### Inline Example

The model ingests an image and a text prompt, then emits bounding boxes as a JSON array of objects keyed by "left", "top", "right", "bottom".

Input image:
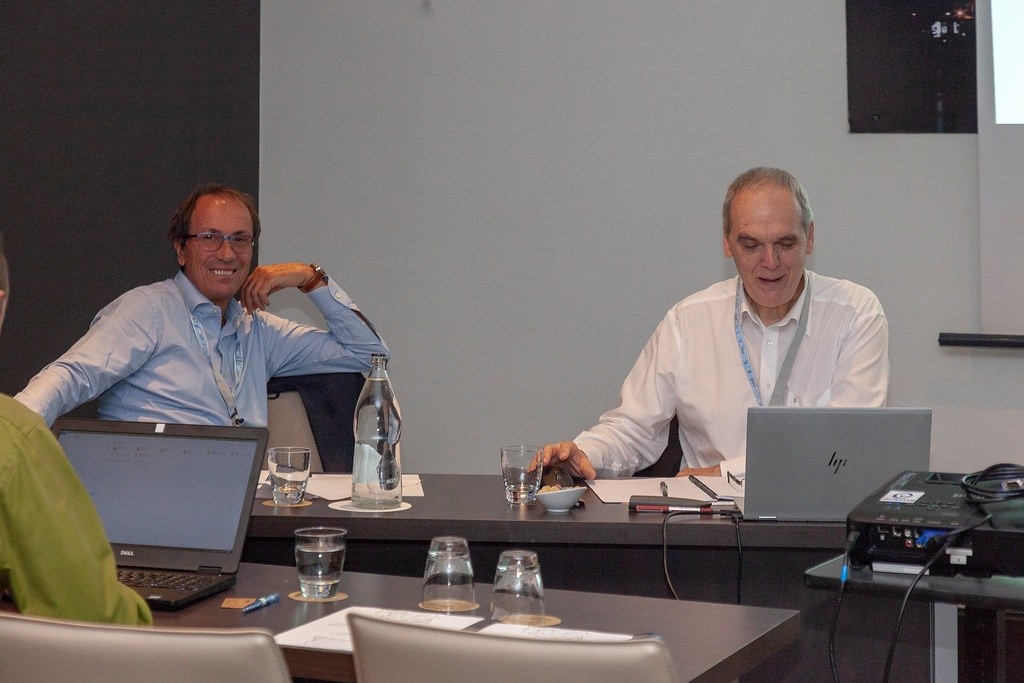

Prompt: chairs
[
  {"left": 0, "top": 610, "right": 294, "bottom": 683},
  {"left": 347, "top": 613, "right": 677, "bottom": 683}
]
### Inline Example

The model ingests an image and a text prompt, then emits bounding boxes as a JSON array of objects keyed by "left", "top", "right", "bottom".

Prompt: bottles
[{"left": 351, "top": 353, "right": 402, "bottom": 509}]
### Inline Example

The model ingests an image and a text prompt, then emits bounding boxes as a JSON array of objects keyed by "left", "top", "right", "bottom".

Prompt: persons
[
  {"left": 527, "top": 166, "right": 890, "bottom": 480},
  {"left": 14, "top": 183, "right": 391, "bottom": 426},
  {"left": 0, "top": 251, "right": 152, "bottom": 626}
]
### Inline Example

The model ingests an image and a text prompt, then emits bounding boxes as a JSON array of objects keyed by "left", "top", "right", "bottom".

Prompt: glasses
[{"left": 180, "top": 232, "right": 256, "bottom": 253}]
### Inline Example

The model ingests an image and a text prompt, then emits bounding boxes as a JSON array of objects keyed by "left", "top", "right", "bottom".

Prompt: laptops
[
  {"left": 734, "top": 407, "right": 933, "bottom": 521},
  {"left": 0, "top": 417, "right": 270, "bottom": 613}
]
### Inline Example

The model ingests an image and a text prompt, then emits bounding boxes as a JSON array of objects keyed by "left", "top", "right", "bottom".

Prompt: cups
[
  {"left": 500, "top": 445, "right": 544, "bottom": 504},
  {"left": 423, "top": 537, "right": 474, "bottom": 611},
  {"left": 266, "top": 447, "right": 311, "bottom": 506},
  {"left": 294, "top": 526, "right": 347, "bottom": 599},
  {"left": 490, "top": 549, "right": 544, "bottom": 626}
]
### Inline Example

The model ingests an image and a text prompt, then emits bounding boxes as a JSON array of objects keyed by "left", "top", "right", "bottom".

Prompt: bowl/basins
[{"left": 533, "top": 487, "right": 588, "bottom": 512}]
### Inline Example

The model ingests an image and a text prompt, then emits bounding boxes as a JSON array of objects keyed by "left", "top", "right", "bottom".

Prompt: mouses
[{"left": 532, "top": 465, "right": 574, "bottom": 489}]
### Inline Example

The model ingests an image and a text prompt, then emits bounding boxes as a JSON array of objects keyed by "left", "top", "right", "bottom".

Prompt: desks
[{"left": 149, "top": 472, "right": 1024, "bottom": 683}]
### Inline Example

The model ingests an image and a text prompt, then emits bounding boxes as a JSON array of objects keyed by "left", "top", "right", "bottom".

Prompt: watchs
[{"left": 302, "top": 263, "right": 326, "bottom": 293}]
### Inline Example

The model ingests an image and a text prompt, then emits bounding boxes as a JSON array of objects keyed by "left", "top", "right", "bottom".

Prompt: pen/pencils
[
  {"left": 660, "top": 481, "right": 668, "bottom": 497},
  {"left": 242, "top": 592, "right": 280, "bottom": 614},
  {"left": 689, "top": 475, "right": 720, "bottom": 501},
  {"left": 636, "top": 505, "right": 712, "bottom": 513}
]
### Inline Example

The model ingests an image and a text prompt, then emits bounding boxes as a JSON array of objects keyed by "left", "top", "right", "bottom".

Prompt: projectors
[{"left": 847, "top": 471, "right": 1024, "bottom": 580}]
